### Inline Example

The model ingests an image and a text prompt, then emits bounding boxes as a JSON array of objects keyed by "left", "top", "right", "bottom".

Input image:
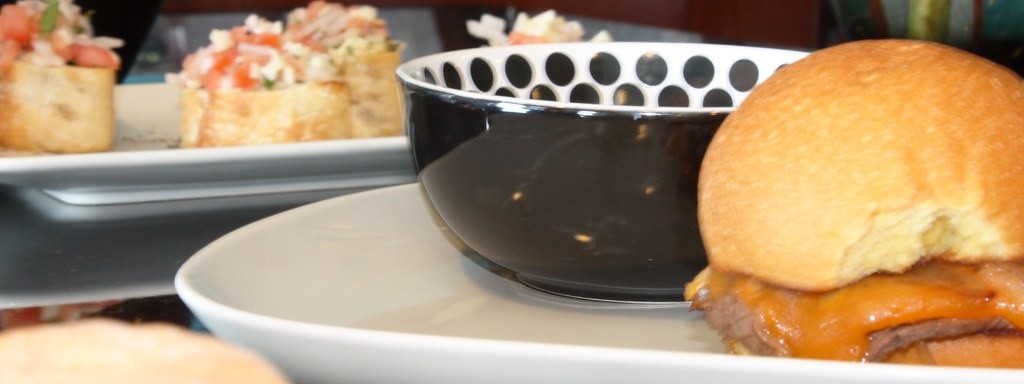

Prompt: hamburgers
[{"left": 682, "top": 38, "right": 1024, "bottom": 374}]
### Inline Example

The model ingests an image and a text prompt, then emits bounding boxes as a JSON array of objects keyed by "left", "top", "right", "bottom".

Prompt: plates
[
  {"left": 122, "top": 9, "right": 444, "bottom": 91},
  {"left": 173, "top": 183, "right": 1024, "bottom": 384},
  {"left": 0, "top": 16, "right": 708, "bottom": 206}
]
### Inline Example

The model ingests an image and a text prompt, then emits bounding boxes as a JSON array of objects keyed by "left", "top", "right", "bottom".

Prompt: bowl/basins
[{"left": 394, "top": 41, "right": 812, "bottom": 305}]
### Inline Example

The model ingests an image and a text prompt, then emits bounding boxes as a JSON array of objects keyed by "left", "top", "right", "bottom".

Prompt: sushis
[{"left": 0, "top": 0, "right": 617, "bottom": 151}]
